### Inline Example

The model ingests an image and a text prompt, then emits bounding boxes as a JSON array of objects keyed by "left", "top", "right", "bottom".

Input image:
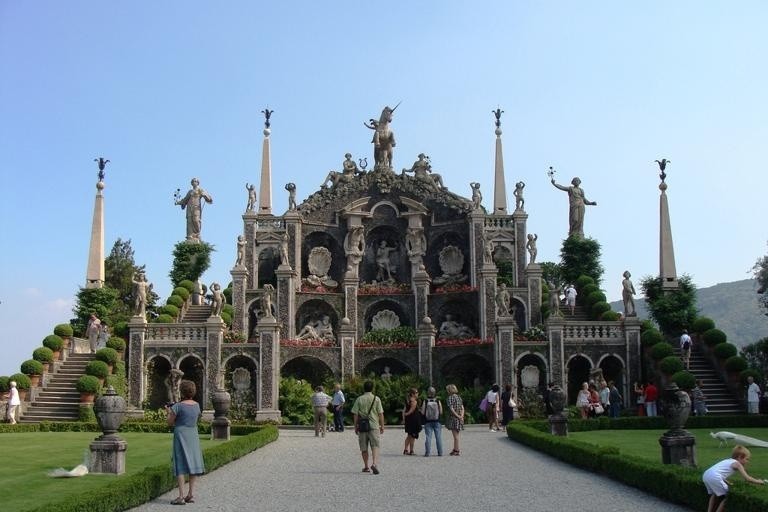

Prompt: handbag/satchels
[
  {"left": 593, "top": 404, "right": 605, "bottom": 414},
  {"left": 357, "top": 417, "right": 370, "bottom": 433},
  {"left": 508, "top": 392, "right": 517, "bottom": 407},
  {"left": 479, "top": 398, "right": 489, "bottom": 412}
]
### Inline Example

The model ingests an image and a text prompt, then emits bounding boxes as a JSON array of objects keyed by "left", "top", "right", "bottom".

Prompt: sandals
[
  {"left": 170, "top": 495, "right": 195, "bottom": 505},
  {"left": 362, "top": 464, "right": 380, "bottom": 474},
  {"left": 449, "top": 450, "right": 461, "bottom": 456},
  {"left": 403, "top": 450, "right": 415, "bottom": 455}
]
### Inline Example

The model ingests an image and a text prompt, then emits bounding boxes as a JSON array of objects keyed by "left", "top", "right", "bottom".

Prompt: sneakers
[{"left": 489, "top": 427, "right": 507, "bottom": 433}]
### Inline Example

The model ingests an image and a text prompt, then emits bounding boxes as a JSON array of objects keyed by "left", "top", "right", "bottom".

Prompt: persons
[
  {"left": 376, "top": 241, "right": 397, "bottom": 280},
  {"left": 440, "top": 315, "right": 474, "bottom": 337},
  {"left": 403, "top": 154, "right": 448, "bottom": 195},
  {"left": 513, "top": 181, "right": 525, "bottom": 210},
  {"left": 495, "top": 284, "right": 511, "bottom": 317},
  {"left": 86, "top": 314, "right": 101, "bottom": 354},
  {"left": 747, "top": 376, "right": 761, "bottom": 415},
  {"left": 176, "top": 178, "right": 212, "bottom": 239},
  {"left": 482, "top": 230, "right": 494, "bottom": 263},
  {"left": 622, "top": 271, "right": 636, "bottom": 316},
  {"left": 131, "top": 272, "right": 149, "bottom": 317},
  {"left": 285, "top": 183, "right": 297, "bottom": 212},
  {"left": 3, "top": 382, "right": 20, "bottom": 425},
  {"left": 363, "top": 120, "right": 379, "bottom": 143},
  {"left": 99, "top": 321, "right": 111, "bottom": 349},
  {"left": 702, "top": 445, "right": 765, "bottom": 512},
  {"left": 164, "top": 369, "right": 185, "bottom": 404},
  {"left": 278, "top": 234, "right": 290, "bottom": 265},
  {"left": 499, "top": 383, "right": 517, "bottom": 431},
  {"left": 544, "top": 382, "right": 555, "bottom": 419},
  {"left": 296, "top": 316, "right": 332, "bottom": 340},
  {"left": 526, "top": 234, "right": 537, "bottom": 263},
  {"left": 164, "top": 380, "right": 205, "bottom": 505},
  {"left": 262, "top": 284, "right": 274, "bottom": 317},
  {"left": 484, "top": 383, "right": 500, "bottom": 432},
  {"left": 576, "top": 379, "right": 658, "bottom": 420},
  {"left": 551, "top": 177, "right": 597, "bottom": 237},
  {"left": 679, "top": 329, "right": 693, "bottom": 370},
  {"left": 312, "top": 380, "right": 464, "bottom": 474},
  {"left": 209, "top": 282, "right": 226, "bottom": 316},
  {"left": 235, "top": 235, "right": 247, "bottom": 267},
  {"left": 693, "top": 379, "right": 707, "bottom": 417},
  {"left": 246, "top": 183, "right": 257, "bottom": 212},
  {"left": 562, "top": 281, "right": 577, "bottom": 317},
  {"left": 320, "top": 153, "right": 365, "bottom": 190},
  {"left": 470, "top": 183, "right": 482, "bottom": 209},
  {"left": 381, "top": 366, "right": 392, "bottom": 386},
  {"left": 548, "top": 284, "right": 562, "bottom": 316}
]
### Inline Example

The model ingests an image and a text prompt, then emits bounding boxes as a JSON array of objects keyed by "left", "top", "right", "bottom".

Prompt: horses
[{"left": 373, "top": 106, "right": 396, "bottom": 169}]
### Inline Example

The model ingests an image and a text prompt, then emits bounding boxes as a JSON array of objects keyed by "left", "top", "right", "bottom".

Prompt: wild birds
[{"left": 709, "top": 431, "right": 768, "bottom": 448}]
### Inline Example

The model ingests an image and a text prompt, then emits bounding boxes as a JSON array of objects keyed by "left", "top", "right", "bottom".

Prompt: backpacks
[
  {"left": 425, "top": 398, "right": 440, "bottom": 422},
  {"left": 683, "top": 341, "right": 690, "bottom": 350}
]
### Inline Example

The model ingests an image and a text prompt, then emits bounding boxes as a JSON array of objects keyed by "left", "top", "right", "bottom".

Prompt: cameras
[{"left": 164, "top": 402, "right": 175, "bottom": 409}]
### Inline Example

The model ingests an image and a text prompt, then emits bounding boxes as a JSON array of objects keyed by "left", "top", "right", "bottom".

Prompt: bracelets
[{"left": 167, "top": 413, "right": 170, "bottom": 414}]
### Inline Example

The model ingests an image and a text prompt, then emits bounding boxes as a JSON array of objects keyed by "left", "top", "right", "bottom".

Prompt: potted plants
[
  {"left": 592, "top": 301, "right": 745, "bottom": 399},
  {"left": 0, "top": 314, "right": 173, "bottom": 403}
]
